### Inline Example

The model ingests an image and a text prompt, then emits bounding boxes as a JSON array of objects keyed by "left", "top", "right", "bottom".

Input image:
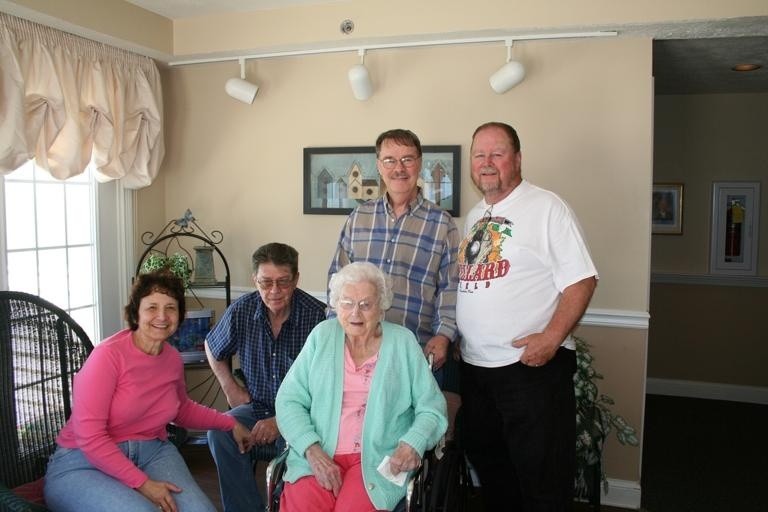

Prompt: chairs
[
  {"left": 0, "top": 291, "right": 187, "bottom": 512},
  {"left": 229, "top": 369, "right": 280, "bottom": 475}
]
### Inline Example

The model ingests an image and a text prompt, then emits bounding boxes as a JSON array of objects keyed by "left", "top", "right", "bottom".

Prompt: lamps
[
  {"left": 349, "top": 49, "right": 373, "bottom": 100},
  {"left": 489, "top": 40, "right": 526, "bottom": 94},
  {"left": 224, "top": 59, "right": 259, "bottom": 105}
]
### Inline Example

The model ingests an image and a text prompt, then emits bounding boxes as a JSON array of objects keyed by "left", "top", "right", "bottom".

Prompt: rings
[{"left": 262, "top": 436, "right": 270, "bottom": 442}]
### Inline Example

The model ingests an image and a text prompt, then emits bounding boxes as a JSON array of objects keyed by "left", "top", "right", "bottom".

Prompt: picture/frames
[
  {"left": 303, "top": 145, "right": 461, "bottom": 217},
  {"left": 708, "top": 181, "right": 761, "bottom": 276},
  {"left": 652, "top": 182, "right": 684, "bottom": 236}
]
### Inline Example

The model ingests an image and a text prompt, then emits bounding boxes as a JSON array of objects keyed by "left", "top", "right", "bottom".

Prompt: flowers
[{"left": 139, "top": 252, "right": 206, "bottom": 308}]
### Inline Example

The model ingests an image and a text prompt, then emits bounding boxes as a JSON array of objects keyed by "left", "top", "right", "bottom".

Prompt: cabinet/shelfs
[{"left": 131, "top": 208, "right": 231, "bottom": 452}]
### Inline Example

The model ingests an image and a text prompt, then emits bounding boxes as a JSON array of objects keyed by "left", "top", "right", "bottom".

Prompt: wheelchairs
[{"left": 266, "top": 353, "right": 436, "bottom": 512}]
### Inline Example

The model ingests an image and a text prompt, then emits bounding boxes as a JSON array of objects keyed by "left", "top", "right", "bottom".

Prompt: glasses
[
  {"left": 377, "top": 156, "right": 422, "bottom": 169},
  {"left": 256, "top": 279, "right": 296, "bottom": 289},
  {"left": 337, "top": 296, "right": 376, "bottom": 311}
]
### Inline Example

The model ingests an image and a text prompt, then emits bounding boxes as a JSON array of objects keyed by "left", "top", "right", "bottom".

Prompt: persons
[
  {"left": 46, "top": 273, "right": 255, "bottom": 511},
  {"left": 458, "top": 122, "right": 598, "bottom": 512},
  {"left": 205, "top": 243, "right": 326, "bottom": 511},
  {"left": 275, "top": 261, "right": 447, "bottom": 512},
  {"left": 323, "top": 129, "right": 456, "bottom": 373}
]
